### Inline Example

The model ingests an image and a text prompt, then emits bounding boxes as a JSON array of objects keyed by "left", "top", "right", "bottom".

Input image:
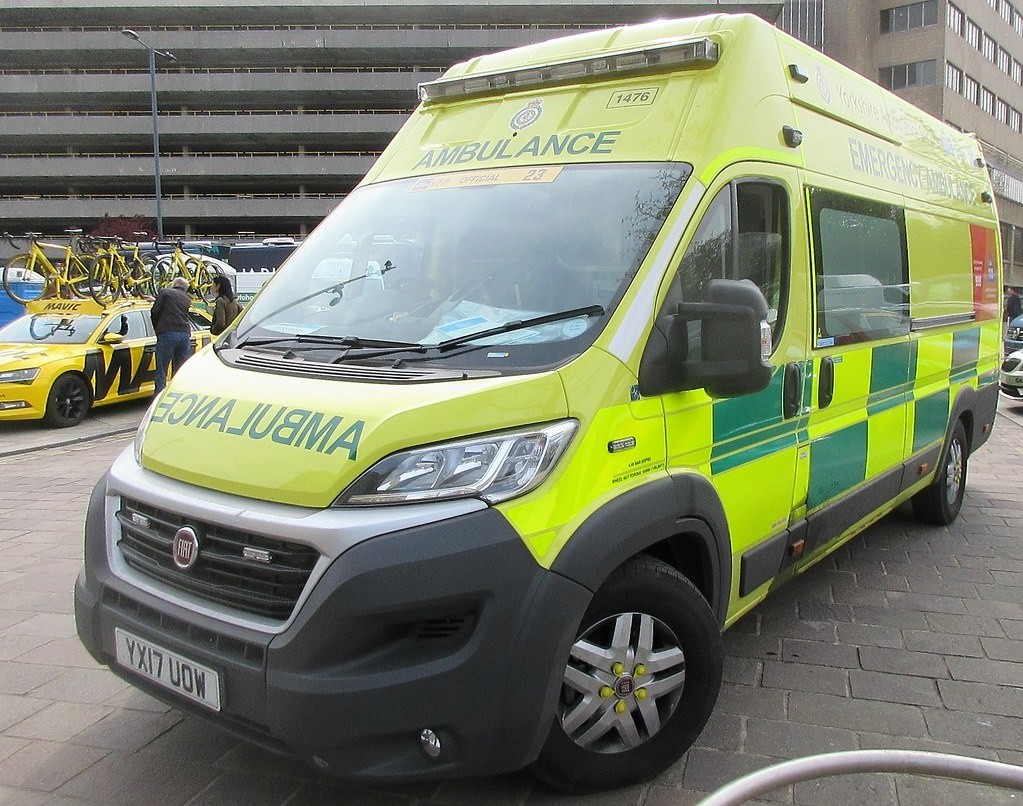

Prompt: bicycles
[{"left": 0, "top": 229, "right": 224, "bottom": 306}]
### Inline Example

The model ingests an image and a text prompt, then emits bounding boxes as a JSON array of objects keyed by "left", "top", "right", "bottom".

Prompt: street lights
[{"left": 123, "top": 30, "right": 177, "bottom": 243}]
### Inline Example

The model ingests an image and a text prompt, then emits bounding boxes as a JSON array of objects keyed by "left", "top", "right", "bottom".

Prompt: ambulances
[{"left": 74, "top": 12, "right": 1004, "bottom": 791}]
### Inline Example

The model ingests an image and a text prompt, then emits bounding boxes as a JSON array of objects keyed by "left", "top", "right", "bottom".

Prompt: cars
[{"left": 0, "top": 304, "right": 222, "bottom": 428}]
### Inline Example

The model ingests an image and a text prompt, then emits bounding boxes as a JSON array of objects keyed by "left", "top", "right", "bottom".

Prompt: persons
[
  {"left": 143, "top": 277, "right": 192, "bottom": 409},
  {"left": 210, "top": 276, "right": 244, "bottom": 341},
  {"left": 1006, "top": 287, "right": 1022, "bottom": 326}
]
